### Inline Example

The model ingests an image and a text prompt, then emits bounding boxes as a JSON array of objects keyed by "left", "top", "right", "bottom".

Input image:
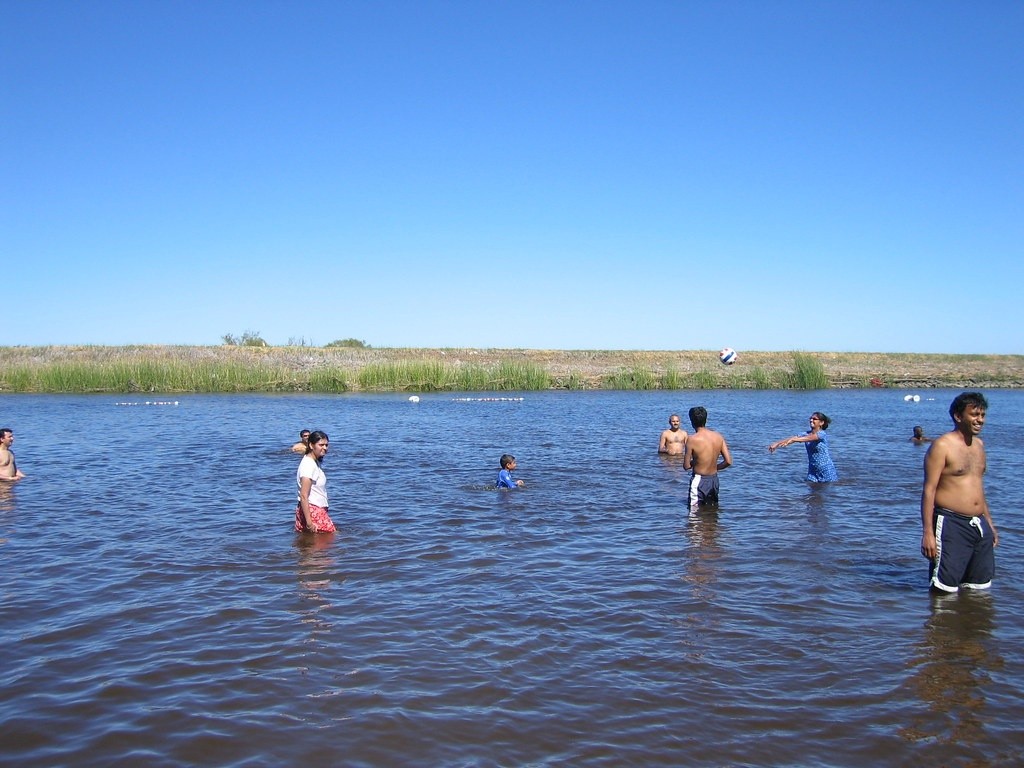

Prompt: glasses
[{"left": 810, "top": 417, "right": 821, "bottom": 420}]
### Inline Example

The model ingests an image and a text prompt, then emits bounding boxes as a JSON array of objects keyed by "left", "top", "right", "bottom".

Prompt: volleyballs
[{"left": 719, "top": 348, "right": 737, "bottom": 365}]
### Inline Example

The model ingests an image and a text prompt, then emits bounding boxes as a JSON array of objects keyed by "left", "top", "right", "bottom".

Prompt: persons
[
  {"left": 0, "top": 428, "right": 25, "bottom": 481},
  {"left": 681, "top": 406, "right": 732, "bottom": 512},
  {"left": 921, "top": 391, "right": 999, "bottom": 592},
  {"left": 294, "top": 429, "right": 335, "bottom": 534},
  {"left": 497, "top": 454, "right": 524, "bottom": 489},
  {"left": 658, "top": 414, "right": 688, "bottom": 454},
  {"left": 769, "top": 411, "right": 839, "bottom": 483},
  {"left": 909, "top": 425, "right": 935, "bottom": 444},
  {"left": 292, "top": 429, "right": 310, "bottom": 454}
]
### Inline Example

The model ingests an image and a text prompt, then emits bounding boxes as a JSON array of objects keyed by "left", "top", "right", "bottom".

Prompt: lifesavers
[{"left": 871, "top": 378, "right": 881, "bottom": 387}]
